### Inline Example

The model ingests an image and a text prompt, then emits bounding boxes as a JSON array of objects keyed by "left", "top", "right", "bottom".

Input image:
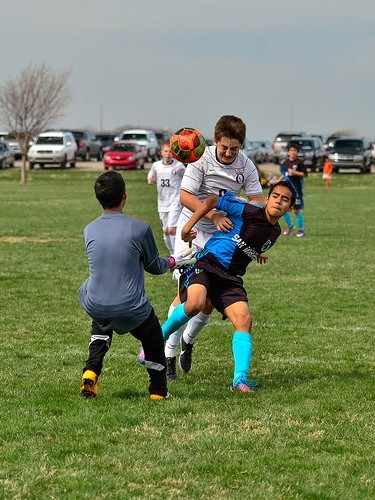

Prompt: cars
[
  {"left": 0, "top": 131, "right": 22, "bottom": 161},
  {"left": 103, "top": 141, "right": 145, "bottom": 171},
  {"left": 91, "top": 133, "right": 116, "bottom": 149},
  {"left": 0, "top": 140, "right": 15, "bottom": 169}
]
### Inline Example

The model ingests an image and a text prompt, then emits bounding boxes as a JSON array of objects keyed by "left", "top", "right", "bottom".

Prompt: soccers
[{"left": 170, "top": 127, "right": 206, "bottom": 163}]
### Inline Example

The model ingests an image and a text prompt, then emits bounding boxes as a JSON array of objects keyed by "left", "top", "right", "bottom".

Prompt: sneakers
[
  {"left": 296, "top": 231, "right": 305, "bottom": 237},
  {"left": 179, "top": 335, "right": 194, "bottom": 372},
  {"left": 283, "top": 226, "right": 296, "bottom": 236},
  {"left": 165, "top": 356, "right": 178, "bottom": 380},
  {"left": 150, "top": 391, "right": 172, "bottom": 400},
  {"left": 139, "top": 348, "right": 145, "bottom": 364},
  {"left": 229, "top": 382, "right": 254, "bottom": 393}
]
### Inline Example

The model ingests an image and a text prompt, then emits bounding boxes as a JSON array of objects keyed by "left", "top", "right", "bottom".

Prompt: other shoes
[{"left": 81, "top": 370, "right": 97, "bottom": 398}]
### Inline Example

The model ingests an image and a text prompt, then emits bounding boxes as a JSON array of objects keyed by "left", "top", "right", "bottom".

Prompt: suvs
[
  {"left": 271, "top": 132, "right": 308, "bottom": 165},
  {"left": 114, "top": 129, "right": 158, "bottom": 163},
  {"left": 70, "top": 130, "right": 104, "bottom": 163},
  {"left": 26, "top": 131, "right": 79, "bottom": 170},
  {"left": 326, "top": 137, "right": 372, "bottom": 174},
  {"left": 279, "top": 137, "right": 325, "bottom": 174},
  {"left": 153, "top": 132, "right": 166, "bottom": 150}
]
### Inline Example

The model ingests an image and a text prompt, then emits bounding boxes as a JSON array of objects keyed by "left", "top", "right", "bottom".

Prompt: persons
[
  {"left": 322, "top": 156, "right": 333, "bottom": 188},
  {"left": 275, "top": 146, "right": 307, "bottom": 238},
  {"left": 148, "top": 140, "right": 186, "bottom": 278},
  {"left": 76, "top": 171, "right": 196, "bottom": 401},
  {"left": 164, "top": 116, "right": 264, "bottom": 378},
  {"left": 138, "top": 180, "right": 297, "bottom": 393}
]
